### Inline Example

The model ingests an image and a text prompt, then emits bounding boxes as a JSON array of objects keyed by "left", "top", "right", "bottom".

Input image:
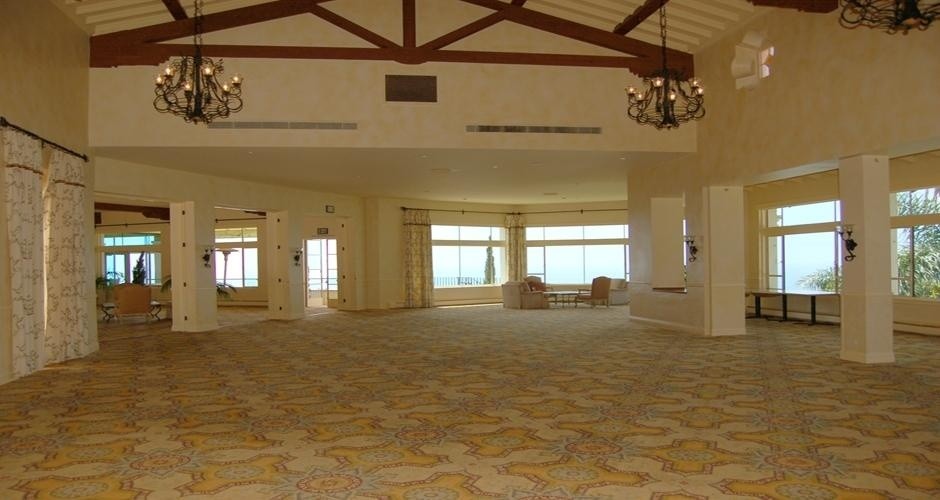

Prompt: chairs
[
  {"left": 112, "top": 285, "right": 151, "bottom": 326},
  {"left": 576, "top": 275, "right": 611, "bottom": 310},
  {"left": 501, "top": 279, "right": 547, "bottom": 309},
  {"left": 608, "top": 279, "right": 629, "bottom": 305},
  {"left": 524, "top": 277, "right": 558, "bottom": 303}
]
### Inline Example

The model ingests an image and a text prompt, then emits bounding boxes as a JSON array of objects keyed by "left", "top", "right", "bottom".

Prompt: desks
[
  {"left": 544, "top": 291, "right": 588, "bottom": 308},
  {"left": 101, "top": 300, "right": 162, "bottom": 325},
  {"left": 749, "top": 290, "right": 838, "bottom": 327}
]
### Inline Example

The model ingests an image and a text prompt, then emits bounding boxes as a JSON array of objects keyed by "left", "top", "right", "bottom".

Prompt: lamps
[
  {"left": 626, "top": 0, "right": 707, "bottom": 130},
  {"left": 682, "top": 235, "right": 698, "bottom": 262},
  {"left": 835, "top": 222, "right": 860, "bottom": 262},
  {"left": 149, "top": 0, "right": 244, "bottom": 125},
  {"left": 832, "top": 1, "right": 940, "bottom": 38}
]
